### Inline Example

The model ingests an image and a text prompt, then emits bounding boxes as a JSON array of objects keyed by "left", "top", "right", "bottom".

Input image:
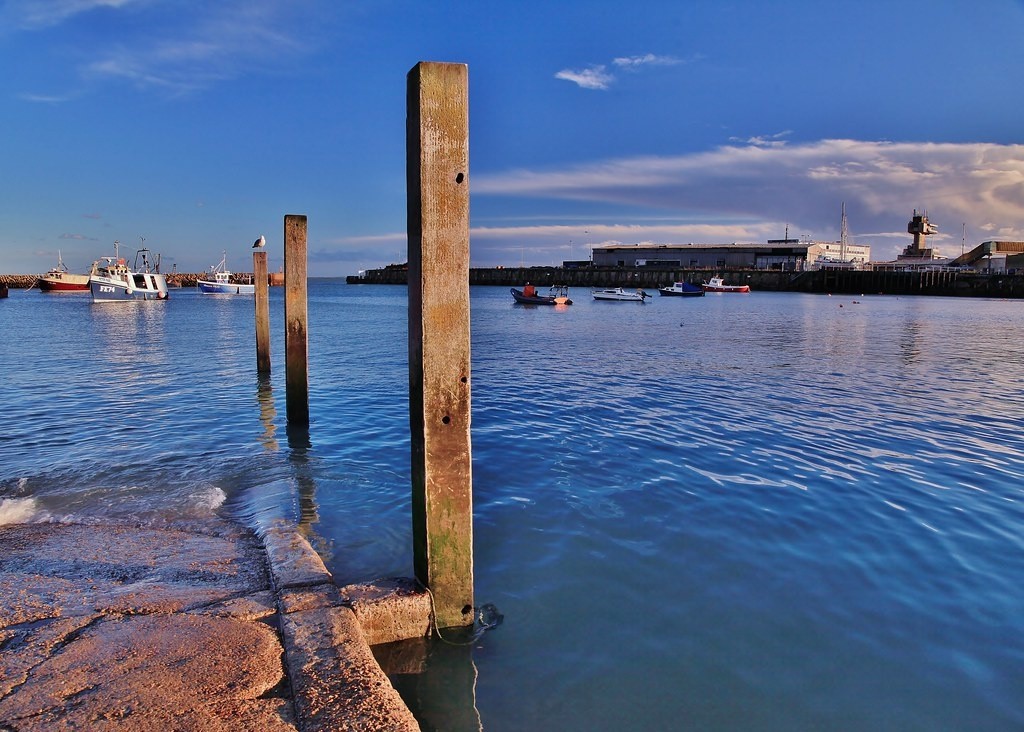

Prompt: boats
[
  {"left": 89, "top": 236, "right": 171, "bottom": 302},
  {"left": 510, "top": 285, "right": 573, "bottom": 306},
  {"left": 658, "top": 281, "right": 705, "bottom": 296},
  {"left": 0, "top": 280, "right": 8, "bottom": 298},
  {"left": 196, "top": 250, "right": 269, "bottom": 294},
  {"left": 590, "top": 287, "right": 652, "bottom": 301},
  {"left": 36, "top": 250, "right": 91, "bottom": 293},
  {"left": 702, "top": 276, "right": 750, "bottom": 292}
]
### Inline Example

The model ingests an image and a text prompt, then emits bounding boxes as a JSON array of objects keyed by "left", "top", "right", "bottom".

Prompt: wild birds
[{"left": 252, "top": 234, "right": 265, "bottom": 251}]
